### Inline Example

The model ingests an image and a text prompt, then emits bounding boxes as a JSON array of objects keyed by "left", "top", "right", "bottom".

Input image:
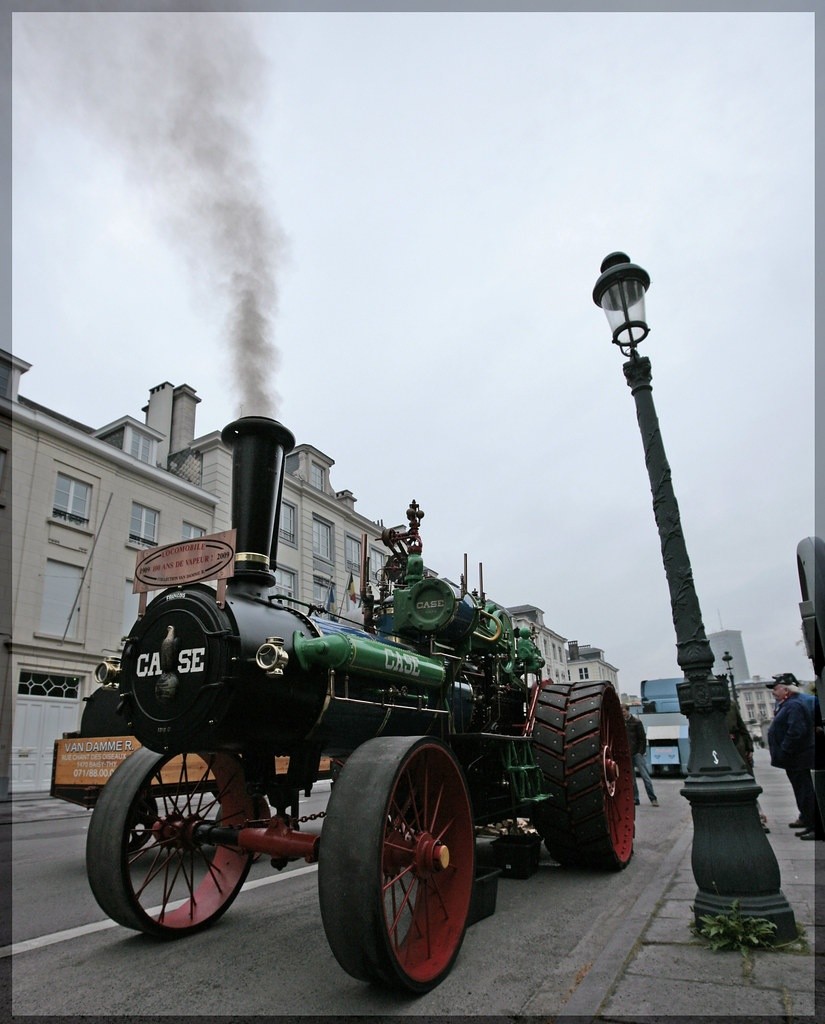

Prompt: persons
[
  {"left": 725, "top": 698, "right": 771, "bottom": 834},
  {"left": 621, "top": 703, "right": 659, "bottom": 807},
  {"left": 765, "top": 672, "right": 823, "bottom": 840}
]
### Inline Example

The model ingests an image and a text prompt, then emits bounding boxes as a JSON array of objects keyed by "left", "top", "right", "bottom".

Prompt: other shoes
[
  {"left": 761, "top": 823, "right": 769, "bottom": 833},
  {"left": 634, "top": 799, "right": 640, "bottom": 804},
  {"left": 789, "top": 819, "right": 806, "bottom": 828},
  {"left": 795, "top": 829, "right": 812, "bottom": 836},
  {"left": 801, "top": 831, "right": 819, "bottom": 840},
  {"left": 651, "top": 799, "right": 659, "bottom": 807}
]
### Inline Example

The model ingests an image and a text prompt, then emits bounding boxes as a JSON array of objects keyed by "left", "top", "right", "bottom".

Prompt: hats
[
  {"left": 766, "top": 677, "right": 791, "bottom": 689},
  {"left": 772, "top": 672, "right": 800, "bottom": 686}
]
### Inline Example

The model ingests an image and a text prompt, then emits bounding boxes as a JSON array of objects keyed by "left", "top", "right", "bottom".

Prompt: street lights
[
  {"left": 598, "top": 252, "right": 805, "bottom": 949},
  {"left": 720, "top": 650, "right": 742, "bottom": 714}
]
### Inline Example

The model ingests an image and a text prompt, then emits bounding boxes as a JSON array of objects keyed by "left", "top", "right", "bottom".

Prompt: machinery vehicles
[
  {"left": 627, "top": 678, "right": 689, "bottom": 778},
  {"left": 49, "top": 411, "right": 639, "bottom": 998}
]
